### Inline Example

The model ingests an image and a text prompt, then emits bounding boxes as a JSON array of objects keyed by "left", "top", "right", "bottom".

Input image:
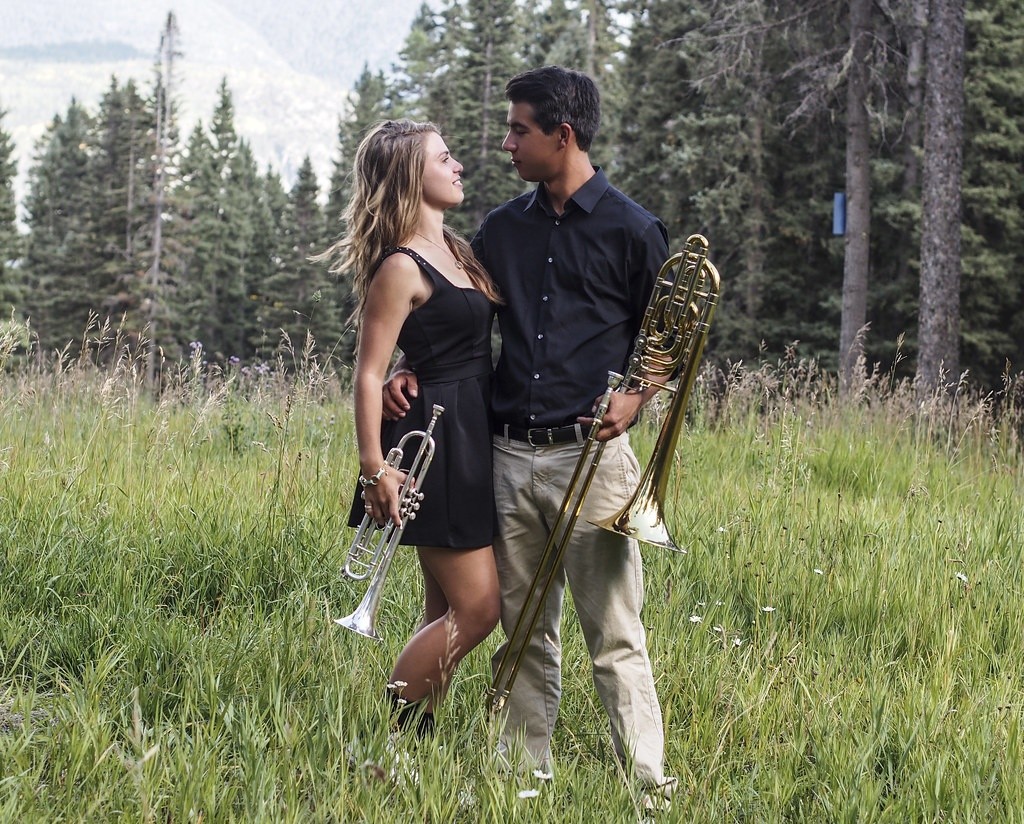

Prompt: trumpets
[{"left": 332, "top": 402, "right": 446, "bottom": 642}]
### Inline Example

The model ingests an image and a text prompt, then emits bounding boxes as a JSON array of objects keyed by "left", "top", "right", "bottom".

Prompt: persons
[
  {"left": 381, "top": 62, "right": 678, "bottom": 824},
  {"left": 302, "top": 117, "right": 504, "bottom": 746}
]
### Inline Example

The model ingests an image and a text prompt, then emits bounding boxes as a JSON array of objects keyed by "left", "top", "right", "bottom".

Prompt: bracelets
[{"left": 359, "top": 460, "right": 389, "bottom": 488}]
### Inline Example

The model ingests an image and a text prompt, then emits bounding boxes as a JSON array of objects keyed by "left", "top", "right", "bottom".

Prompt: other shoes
[{"left": 386, "top": 681, "right": 438, "bottom": 757}]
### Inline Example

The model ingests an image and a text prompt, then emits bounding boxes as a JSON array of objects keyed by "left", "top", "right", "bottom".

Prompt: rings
[{"left": 365, "top": 505, "right": 372, "bottom": 509}]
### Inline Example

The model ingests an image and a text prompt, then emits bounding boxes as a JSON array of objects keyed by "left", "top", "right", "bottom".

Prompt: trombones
[{"left": 486, "top": 233, "right": 722, "bottom": 734}]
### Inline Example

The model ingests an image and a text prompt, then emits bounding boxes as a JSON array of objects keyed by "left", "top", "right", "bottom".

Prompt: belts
[{"left": 493, "top": 421, "right": 591, "bottom": 446}]
